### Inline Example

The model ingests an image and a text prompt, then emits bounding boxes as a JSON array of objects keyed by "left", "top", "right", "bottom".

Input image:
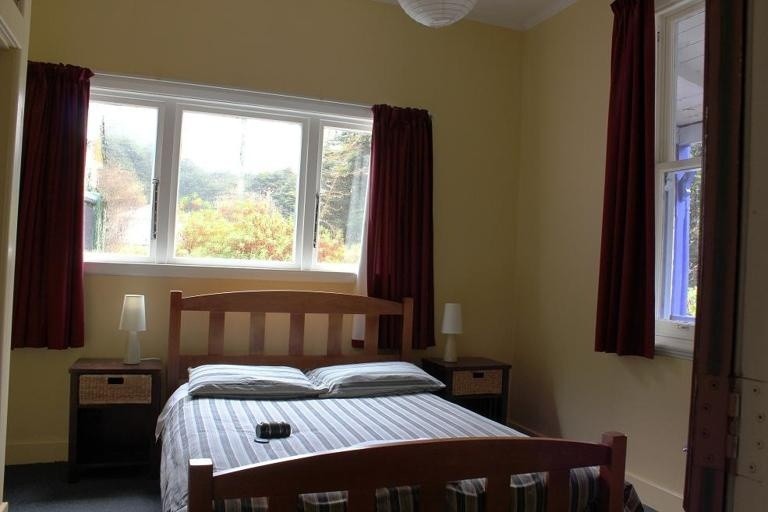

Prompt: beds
[
  {"left": 118, "top": 293, "right": 148, "bottom": 366},
  {"left": 164, "top": 289, "right": 634, "bottom": 512}
]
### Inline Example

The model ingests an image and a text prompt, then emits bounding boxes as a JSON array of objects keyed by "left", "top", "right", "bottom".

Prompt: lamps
[
  {"left": 440, "top": 302, "right": 464, "bottom": 363},
  {"left": 396, "top": 0, "right": 478, "bottom": 29}
]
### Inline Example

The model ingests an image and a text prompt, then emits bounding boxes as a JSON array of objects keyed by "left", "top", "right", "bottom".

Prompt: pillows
[
  {"left": 187, "top": 363, "right": 328, "bottom": 400},
  {"left": 305, "top": 361, "right": 446, "bottom": 397}
]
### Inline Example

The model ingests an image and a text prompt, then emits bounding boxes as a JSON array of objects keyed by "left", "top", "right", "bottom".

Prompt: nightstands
[
  {"left": 420, "top": 355, "right": 512, "bottom": 428},
  {"left": 67, "top": 355, "right": 161, "bottom": 482}
]
